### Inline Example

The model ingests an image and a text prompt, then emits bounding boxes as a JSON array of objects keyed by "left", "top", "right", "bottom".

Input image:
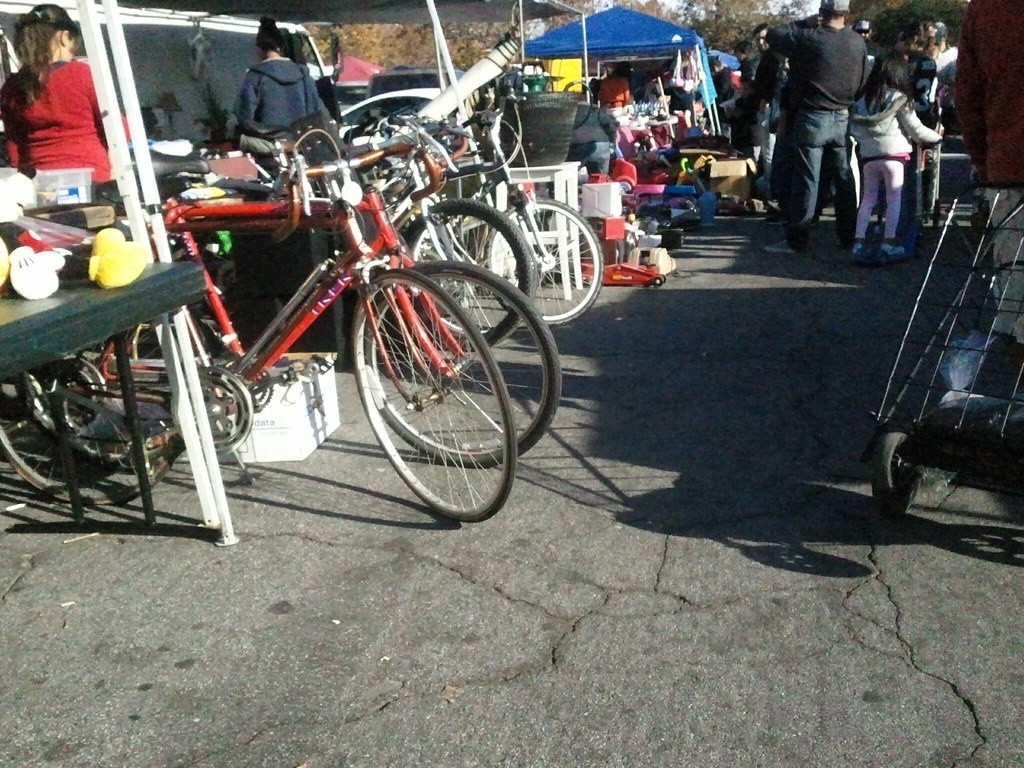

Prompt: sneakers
[{"left": 764, "top": 209, "right": 790, "bottom": 225}]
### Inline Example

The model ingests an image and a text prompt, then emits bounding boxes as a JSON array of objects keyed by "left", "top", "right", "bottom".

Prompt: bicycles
[{"left": 0, "top": 64, "right": 605, "bottom": 526}]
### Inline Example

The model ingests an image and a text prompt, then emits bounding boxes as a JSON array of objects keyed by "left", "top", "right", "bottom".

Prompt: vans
[{"left": 2, "top": 1, "right": 343, "bottom": 171}]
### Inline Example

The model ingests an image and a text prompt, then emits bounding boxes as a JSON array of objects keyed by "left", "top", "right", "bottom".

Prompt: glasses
[{"left": 855, "top": 29, "right": 869, "bottom": 33}]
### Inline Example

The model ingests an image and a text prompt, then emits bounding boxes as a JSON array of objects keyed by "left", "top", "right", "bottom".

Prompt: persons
[
  {"left": 598, "top": 67, "right": 633, "bottom": 112},
  {"left": 0, "top": 2, "right": 131, "bottom": 190},
  {"left": 233, "top": 17, "right": 336, "bottom": 139},
  {"left": 722, "top": 1, "right": 967, "bottom": 263}
]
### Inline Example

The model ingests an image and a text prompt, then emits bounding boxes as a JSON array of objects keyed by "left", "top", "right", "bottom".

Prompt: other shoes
[
  {"left": 851, "top": 242, "right": 866, "bottom": 258},
  {"left": 761, "top": 231, "right": 805, "bottom": 254},
  {"left": 876, "top": 243, "right": 905, "bottom": 259}
]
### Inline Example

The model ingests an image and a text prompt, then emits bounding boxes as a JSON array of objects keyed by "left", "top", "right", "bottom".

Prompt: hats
[
  {"left": 14, "top": 4, "right": 79, "bottom": 36},
  {"left": 820, "top": 0, "right": 850, "bottom": 12},
  {"left": 854, "top": 18, "right": 873, "bottom": 30}
]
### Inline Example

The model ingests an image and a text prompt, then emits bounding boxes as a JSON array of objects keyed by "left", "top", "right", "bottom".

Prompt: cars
[{"left": 337, "top": 87, "right": 440, "bottom": 146}]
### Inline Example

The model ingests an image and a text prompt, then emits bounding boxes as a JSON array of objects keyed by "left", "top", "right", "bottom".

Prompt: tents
[{"left": 522, "top": 6, "right": 718, "bottom": 134}]
